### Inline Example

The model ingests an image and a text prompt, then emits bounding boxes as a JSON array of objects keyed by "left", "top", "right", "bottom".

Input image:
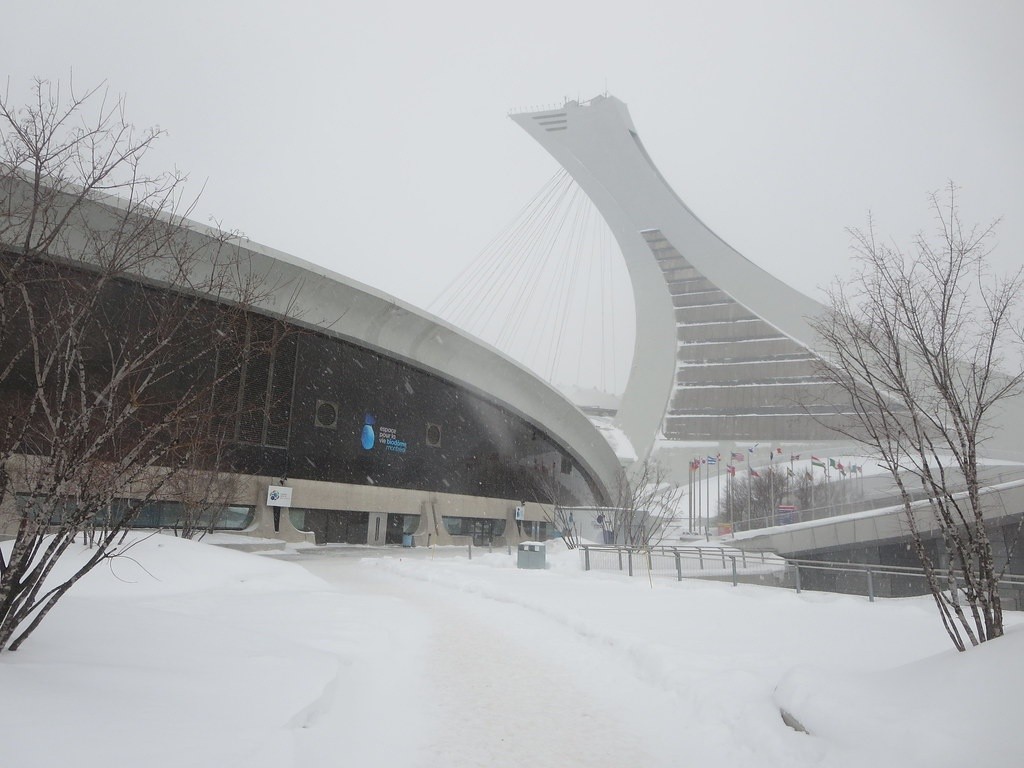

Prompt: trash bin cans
[
  {"left": 402, "top": 533, "right": 412, "bottom": 548},
  {"left": 517, "top": 541, "right": 546, "bottom": 570}
]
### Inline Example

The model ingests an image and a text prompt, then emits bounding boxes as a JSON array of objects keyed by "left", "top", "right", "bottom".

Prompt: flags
[
  {"left": 689, "top": 447, "right": 862, "bottom": 481},
  {"left": 778, "top": 505, "right": 793, "bottom": 513}
]
